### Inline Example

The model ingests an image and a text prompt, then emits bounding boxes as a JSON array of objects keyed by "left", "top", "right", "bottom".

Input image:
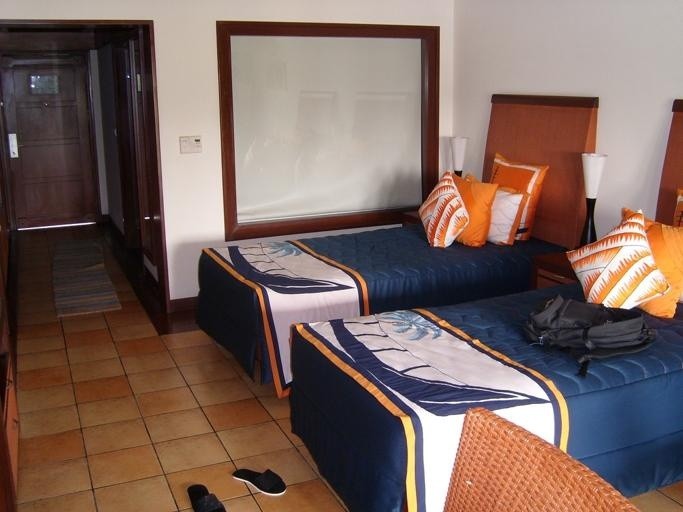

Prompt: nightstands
[
  {"left": 533, "top": 252, "right": 579, "bottom": 290},
  {"left": 400, "top": 205, "right": 422, "bottom": 224}
]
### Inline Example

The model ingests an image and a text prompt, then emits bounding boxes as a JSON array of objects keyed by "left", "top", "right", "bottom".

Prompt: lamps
[
  {"left": 580, "top": 154, "right": 608, "bottom": 249},
  {"left": 448, "top": 137, "right": 467, "bottom": 178}
]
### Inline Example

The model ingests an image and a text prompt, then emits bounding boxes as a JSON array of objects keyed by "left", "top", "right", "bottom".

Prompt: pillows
[
  {"left": 451, "top": 172, "right": 498, "bottom": 248},
  {"left": 418, "top": 169, "right": 469, "bottom": 249},
  {"left": 488, "top": 152, "right": 549, "bottom": 241},
  {"left": 488, "top": 189, "right": 530, "bottom": 246},
  {"left": 621, "top": 204, "right": 682, "bottom": 319},
  {"left": 566, "top": 209, "right": 669, "bottom": 309}
]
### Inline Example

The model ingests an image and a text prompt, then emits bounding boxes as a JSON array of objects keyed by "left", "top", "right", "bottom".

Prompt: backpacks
[{"left": 523, "top": 295, "right": 658, "bottom": 377}]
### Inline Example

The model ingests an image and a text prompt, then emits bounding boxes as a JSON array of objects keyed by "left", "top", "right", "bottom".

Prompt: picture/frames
[{"left": 216, "top": 19, "right": 441, "bottom": 241}]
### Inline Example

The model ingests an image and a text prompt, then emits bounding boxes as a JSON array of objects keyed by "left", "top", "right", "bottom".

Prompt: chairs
[{"left": 442, "top": 407, "right": 641, "bottom": 512}]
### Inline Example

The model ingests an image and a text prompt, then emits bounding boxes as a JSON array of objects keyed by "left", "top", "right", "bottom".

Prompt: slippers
[
  {"left": 187, "top": 484, "right": 226, "bottom": 512},
  {"left": 232, "top": 469, "right": 287, "bottom": 496}
]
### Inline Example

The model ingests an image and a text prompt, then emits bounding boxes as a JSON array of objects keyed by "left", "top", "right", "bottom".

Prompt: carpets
[{"left": 49, "top": 236, "right": 123, "bottom": 319}]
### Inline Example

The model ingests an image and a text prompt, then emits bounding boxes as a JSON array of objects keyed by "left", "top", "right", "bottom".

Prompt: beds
[
  {"left": 197, "top": 95, "right": 597, "bottom": 397},
  {"left": 288, "top": 98, "right": 682, "bottom": 512}
]
